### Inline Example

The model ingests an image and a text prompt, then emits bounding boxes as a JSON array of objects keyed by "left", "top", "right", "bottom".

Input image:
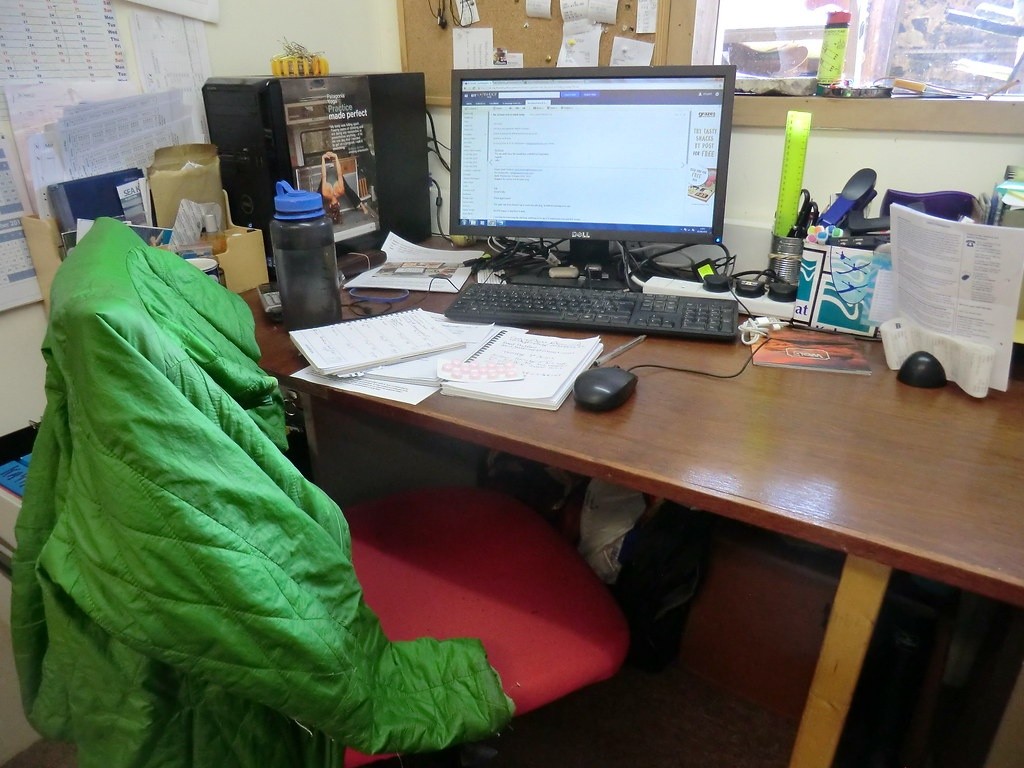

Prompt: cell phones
[{"left": 257, "top": 282, "right": 283, "bottom": 314}]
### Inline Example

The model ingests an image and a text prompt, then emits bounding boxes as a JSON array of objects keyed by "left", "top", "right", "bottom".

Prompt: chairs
[{"left": 12, "top": 216, "right": 631, "bottom": 768}]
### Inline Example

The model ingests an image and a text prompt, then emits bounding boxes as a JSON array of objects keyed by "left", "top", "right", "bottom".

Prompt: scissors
[{"left": 794, "top": 189, "right": 819, "bottom": 239}]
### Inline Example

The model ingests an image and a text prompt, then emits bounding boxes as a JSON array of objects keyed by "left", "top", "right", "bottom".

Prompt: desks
[{"left": 239, "top": 238, "right": 1024, "bottom": 768}]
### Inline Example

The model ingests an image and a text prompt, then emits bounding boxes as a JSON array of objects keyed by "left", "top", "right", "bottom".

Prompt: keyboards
[{"left": 445, "top": 282, "right": 738, "bottom": 342}]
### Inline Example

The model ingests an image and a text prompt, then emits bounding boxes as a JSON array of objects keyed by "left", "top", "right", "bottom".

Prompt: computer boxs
[{"left": 201, "top": 70, "right": 431, "bottom": 285}]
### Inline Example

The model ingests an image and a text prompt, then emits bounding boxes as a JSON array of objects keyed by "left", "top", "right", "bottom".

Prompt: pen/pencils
[{"left": 593, "top": 335, "right": 647, "bottom": 367}]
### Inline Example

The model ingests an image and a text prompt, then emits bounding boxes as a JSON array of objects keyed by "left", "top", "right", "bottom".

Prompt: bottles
[
  {"left": 202, "top": 214, "right": 227, "bottom": 255},
  {"left": 272, "top": 180, "right": 342, "bottom": 331},
  {"left": 817, "top": 11, "right": 851, "bottom": 94},
  {"left": 988, "top": 165, "right": 1024, "bottom": 226},
  {"left": 209, "top": 256, "right": 226, "bottom": 289}
]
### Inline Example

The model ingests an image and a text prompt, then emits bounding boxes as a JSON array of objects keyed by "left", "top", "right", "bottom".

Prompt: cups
[
  {"left": 769, "top": 233, "right": 803, "bottom": 293},
  {"left": 183, "top": 258, "right": 219, "bottom": 285}
]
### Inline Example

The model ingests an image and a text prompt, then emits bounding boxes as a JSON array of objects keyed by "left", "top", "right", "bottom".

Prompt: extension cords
[{"left": 643, "top": 276, "right": 795, "bottom": 320}]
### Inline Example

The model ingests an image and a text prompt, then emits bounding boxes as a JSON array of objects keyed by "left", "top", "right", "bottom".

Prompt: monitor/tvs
[{"left": 448, "top": 65, "right": 737, "bottom": 291}]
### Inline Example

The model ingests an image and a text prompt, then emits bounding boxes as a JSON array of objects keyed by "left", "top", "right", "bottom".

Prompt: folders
[{"left": 47, "top": 167, "right": 153, "bottom": 232}]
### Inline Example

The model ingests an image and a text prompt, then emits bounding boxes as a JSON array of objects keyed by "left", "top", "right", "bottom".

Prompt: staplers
[{"left": 814, "top": 167, "right": 878, "bottom": 227}]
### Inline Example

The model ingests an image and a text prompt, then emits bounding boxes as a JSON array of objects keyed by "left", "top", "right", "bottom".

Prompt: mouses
[{"left": 573, "top": 364, "right": 638, "bottom": 411}]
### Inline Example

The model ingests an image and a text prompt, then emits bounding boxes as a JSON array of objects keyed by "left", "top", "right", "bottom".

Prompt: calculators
[{"left": 257, "top": 281, "right": 286, "bottom": 324}]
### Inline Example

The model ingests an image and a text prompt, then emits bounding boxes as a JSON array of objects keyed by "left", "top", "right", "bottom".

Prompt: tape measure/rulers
[{"left": 772, "top": 110, "right": 812, "bottom": 239}]
